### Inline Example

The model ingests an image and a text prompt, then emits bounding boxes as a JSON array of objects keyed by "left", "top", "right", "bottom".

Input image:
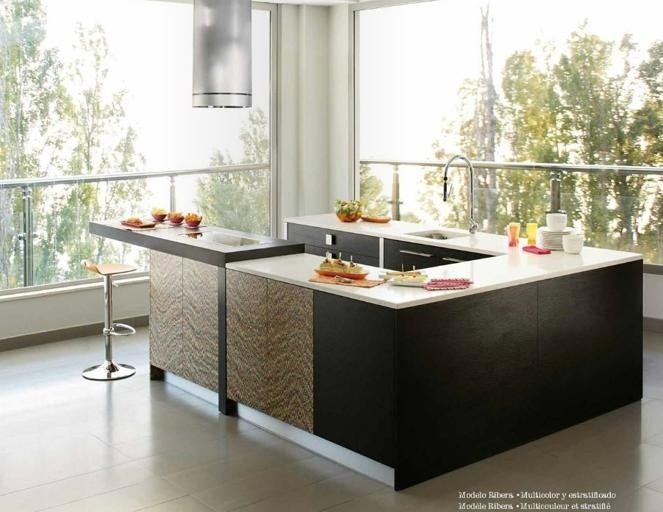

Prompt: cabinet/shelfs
[
  {"left": 383, "top": 238, "right": 491, "bottom": 271},
  {"left": 286, "top": 224, "right": 379, "bottom": 268}
]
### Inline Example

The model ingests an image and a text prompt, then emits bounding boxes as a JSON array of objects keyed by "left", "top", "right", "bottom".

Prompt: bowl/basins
[
  {"left": 546, "top": 212, "right": 568, "bottom": 231},
  {"left": 562, "top": 234, "right": 584, "bottom": 254},
  {"left": 151, "top": 214, "right": 201, "bottom": 226}
]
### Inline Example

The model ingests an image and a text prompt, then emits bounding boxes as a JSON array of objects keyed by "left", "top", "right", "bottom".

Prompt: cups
[{"left": 507, "top": 220, "right": 537, "bottom": 247}]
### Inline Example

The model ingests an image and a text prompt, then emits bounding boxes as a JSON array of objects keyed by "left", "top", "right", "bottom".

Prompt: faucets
[{"left": 443, "top": 154, "right": 478, "bottom": 232}]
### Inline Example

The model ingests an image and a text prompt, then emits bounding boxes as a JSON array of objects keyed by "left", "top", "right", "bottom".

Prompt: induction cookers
[{"left": 177, "top": 230, "right": 262, "bottom": 248}]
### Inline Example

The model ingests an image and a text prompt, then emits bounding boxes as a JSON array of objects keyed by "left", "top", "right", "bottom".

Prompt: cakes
[
  {"left": 317, "top": 257, "right": 362, "bottom": 275},
  {"left": 390, "top": 275, "right": 425, "bottom": 286}
]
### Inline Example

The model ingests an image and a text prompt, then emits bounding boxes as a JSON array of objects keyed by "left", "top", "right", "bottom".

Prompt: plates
[
  {"left": 153, "top": 220, "right": 201, "bottom": 228},
  {"left": 360, "top": 214, "right": 391, "bottom": 224},
  {"left": 120, "top": 219, "right": 156, "bottom": 229},
  {"left": 539, "top": 226, "right": 576, "bottom": 251},
  {"left": 314, "top": 266, "right": 369, "bottom": 280}
]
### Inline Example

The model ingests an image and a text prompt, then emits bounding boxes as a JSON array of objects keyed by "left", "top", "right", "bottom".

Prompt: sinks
[{"left": 404, "top": 228, "right": 469, "bottom": 240}]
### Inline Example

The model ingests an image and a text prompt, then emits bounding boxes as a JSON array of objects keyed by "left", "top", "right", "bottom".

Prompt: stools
[{"left": 80, "top": 260, "right": 139, "bottom": 381}]
